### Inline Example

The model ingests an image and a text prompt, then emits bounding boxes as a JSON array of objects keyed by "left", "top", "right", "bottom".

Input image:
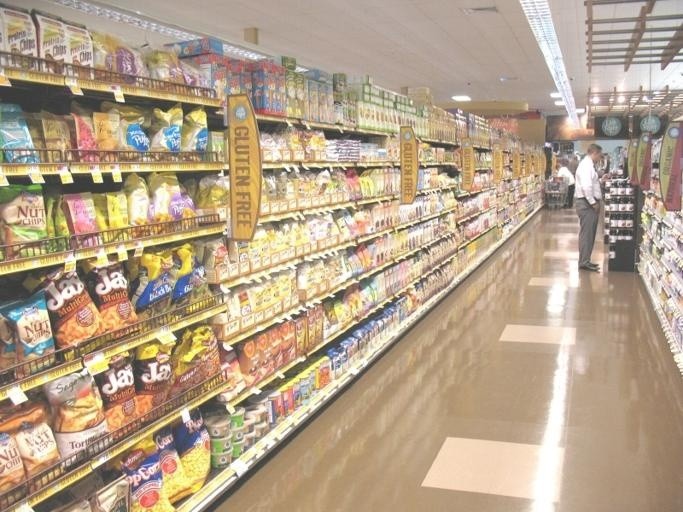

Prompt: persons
[
  {"left": 572, "top": 143, "right": 610, "bottom": 273},
  {"left": 554, "top": 164, "right": 575, "bottom": 209}
]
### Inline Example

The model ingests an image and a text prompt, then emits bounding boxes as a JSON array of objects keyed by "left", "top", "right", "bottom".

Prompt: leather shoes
[{"left": 578, "top": 261, "right": 601, "bottom": 272}]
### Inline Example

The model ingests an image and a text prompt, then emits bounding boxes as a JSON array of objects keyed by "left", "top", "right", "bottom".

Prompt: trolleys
[{"left": 545, "top": 175, "right": 569, "bottom": 208}]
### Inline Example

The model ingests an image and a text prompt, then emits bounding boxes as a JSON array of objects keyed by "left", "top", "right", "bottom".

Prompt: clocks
[{"left": 601, "top": 116, "right": 662, "bottom": 137}]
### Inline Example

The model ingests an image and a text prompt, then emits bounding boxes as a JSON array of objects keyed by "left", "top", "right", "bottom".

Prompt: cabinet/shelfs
[
  {"left": 635, "top": 134, "right": 683, "bottom": 374},
  {"left": 2, "top": 53, "right": 546, "bottom": 512},
  {"left": 601, "top": 180, "right": 636, "bottom": 272}
]
